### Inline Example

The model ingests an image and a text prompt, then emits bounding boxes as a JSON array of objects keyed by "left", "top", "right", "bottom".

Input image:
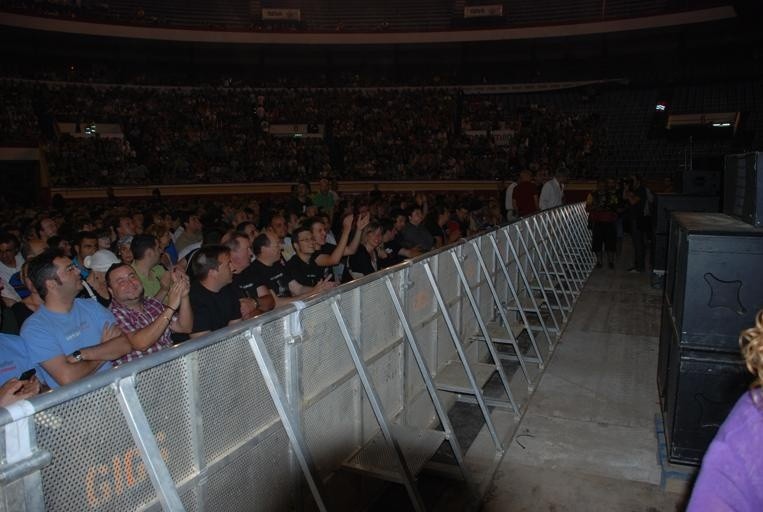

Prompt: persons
[
  {"left": 682, "top": 308, "right": 763, "bottom": 512},
  {"left": 2, "top": 55, "right": 658, "bottom": 433}
]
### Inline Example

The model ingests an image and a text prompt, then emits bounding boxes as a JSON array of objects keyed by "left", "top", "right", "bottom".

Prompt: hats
[{"left": 83, "top": 250, "right": 121, "bottom": 272}]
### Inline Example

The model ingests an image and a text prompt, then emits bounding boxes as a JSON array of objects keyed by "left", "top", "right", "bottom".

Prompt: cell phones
[{"left": 14, "top": 368, "right": 37, "bottom": 395}]
